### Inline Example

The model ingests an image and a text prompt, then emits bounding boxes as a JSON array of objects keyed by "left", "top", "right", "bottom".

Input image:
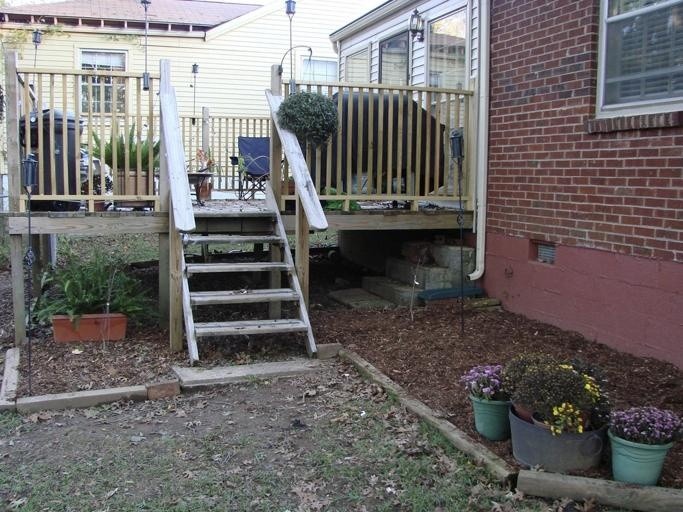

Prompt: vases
[
  {"left": 607, "top": 430, "right": 677, "bottom": 486},
  {"left": 507, "top": 408, "right": 610, "bottom": 472}
]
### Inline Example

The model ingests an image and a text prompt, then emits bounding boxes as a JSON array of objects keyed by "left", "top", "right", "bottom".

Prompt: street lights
[
  {"left": 32, "top": 28, "right": 44, "bottom": 92},
  {"left": 192, "top": 63, "right": 199, "bottom": 116},
  {"left": 285, "top": 0, "right": 296, "bottom": 80},
  {"left": 140, "top": 0, "right": 152, "bottom": 73},
  {"left": 22, "top": 153, "right": 39, "bottom": 397},
  {"left": 449, "top": 129, "right": 465, "bottom": 337}
]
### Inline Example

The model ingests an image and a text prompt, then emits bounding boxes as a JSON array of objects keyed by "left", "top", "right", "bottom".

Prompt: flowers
[{"left": 457, "top": 353, "right": 683, "bottom": 445}]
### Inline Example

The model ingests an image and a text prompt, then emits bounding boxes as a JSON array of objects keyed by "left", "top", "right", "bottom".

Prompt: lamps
[{"left": 410, "top": 7, "right": 425, "bottom": 41}]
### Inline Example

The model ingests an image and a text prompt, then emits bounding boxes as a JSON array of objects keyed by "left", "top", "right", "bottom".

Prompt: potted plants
[
  {"left": 79, "top": 122, "right": 161, "bottom": 212},
  {"left": 281, "top": 175, "right": 295, "bottom": 195},
  {"left": 25, "top": 234, "right": 164, "bottom": 343},
  {"left": 192, "top": 149, "right": 216, "bottom": 200}
]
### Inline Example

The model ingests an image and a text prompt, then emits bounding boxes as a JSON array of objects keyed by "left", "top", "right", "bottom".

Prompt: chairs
[{"left": 230, "top": 136, "right": 269, "bottom": 201}]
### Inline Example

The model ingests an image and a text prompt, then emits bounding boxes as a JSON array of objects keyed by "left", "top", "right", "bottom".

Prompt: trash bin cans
[{"left": 19, "top": 108, "right": 84, "bottom": 211}]
